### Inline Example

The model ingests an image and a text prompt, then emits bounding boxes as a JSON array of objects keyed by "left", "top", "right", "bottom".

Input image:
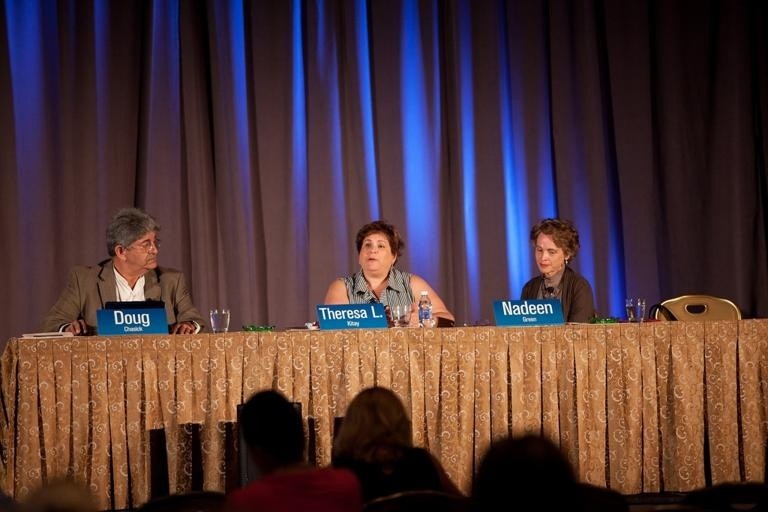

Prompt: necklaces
[{"left": 541, "top": 279, "right": 561, "bottom": 299}]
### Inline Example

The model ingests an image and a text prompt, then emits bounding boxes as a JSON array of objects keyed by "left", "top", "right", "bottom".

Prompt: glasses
[{"left": 125, "top": 239, "right": 160, "bottom": 250}]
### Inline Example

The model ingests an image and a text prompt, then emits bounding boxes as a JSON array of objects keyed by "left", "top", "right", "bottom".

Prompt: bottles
[{"left": 418, "top": 291, "right": 432, "bottom": 327}]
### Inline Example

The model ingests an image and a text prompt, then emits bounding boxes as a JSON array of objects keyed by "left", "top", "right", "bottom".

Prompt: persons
[
  {"left": 468, "top": 435, "right": 630, "bottom": 512},
  {"left": 521, "top": 218, "right": 593, "bottom": 324},
  {"left": 324, "top": 220, "right": 455, "bottom": 329},
  {"left": 223, "top": 391, "right": 361, "bottom": 512},
  {"left": 40, "top": 208, "right": 205, "bottom": 335},
  {"left": 332, "top": 387, "right": 468, "bottom": 512}
]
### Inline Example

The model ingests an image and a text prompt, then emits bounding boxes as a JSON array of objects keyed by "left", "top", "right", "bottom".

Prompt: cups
[
  {"left": 390, "top": 305, "right": 411, "bottom": 327},
  {"left": 209, "top": 309, "right": 231, "bottom": 333},
  {"left": 625, "top": 297, "right": 645, "bottom": 323}
]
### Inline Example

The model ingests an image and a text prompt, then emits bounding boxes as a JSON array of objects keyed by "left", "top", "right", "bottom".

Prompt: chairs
[
  {"left": 655, "top": 294, "right": 741, "bottom": 323},
  {"left": 369, "top": 487, "right": 462, "bottom": 512}
]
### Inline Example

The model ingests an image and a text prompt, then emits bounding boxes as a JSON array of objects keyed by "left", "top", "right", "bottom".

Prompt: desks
[{"left": 1, "top": 318, "right": 768, "bottom": 512}]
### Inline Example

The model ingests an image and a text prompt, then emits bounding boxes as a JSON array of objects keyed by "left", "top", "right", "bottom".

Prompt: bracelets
[
  {"left": 59, "top": 323, "right": 72, "bottom": 332},
  {"left": 180, "top": 320, "right": 200, "bottom": 328}
]
